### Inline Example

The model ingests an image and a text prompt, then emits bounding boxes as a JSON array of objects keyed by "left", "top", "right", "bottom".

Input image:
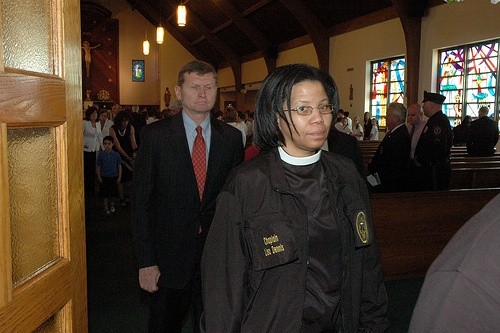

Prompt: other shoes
[
  {"left": 110, "top": 204, "right": 116, "bottom": 213},
  {"left": 120, "top": 199, "right": 126, "bottom": 207},
  {"left": 104, "top": 207, "right": 111, "bottom": 215}
]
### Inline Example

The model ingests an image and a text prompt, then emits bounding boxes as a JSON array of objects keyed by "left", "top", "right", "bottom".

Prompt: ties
[
  {"left": 190, "top": 126, "right": 206, "bottom": 201},
  {"left": 408, "top": 125, "right": 415, "bottom": 144}
]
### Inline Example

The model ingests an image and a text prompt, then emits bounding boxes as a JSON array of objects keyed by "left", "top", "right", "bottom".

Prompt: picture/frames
[{"left": 132, "top": 59, "right": 145, "bottom": 82}]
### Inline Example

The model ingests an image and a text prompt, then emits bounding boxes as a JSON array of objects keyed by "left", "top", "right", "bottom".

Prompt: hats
[{"left": 422, "top": 90, "right": 446, "bottom": 104}]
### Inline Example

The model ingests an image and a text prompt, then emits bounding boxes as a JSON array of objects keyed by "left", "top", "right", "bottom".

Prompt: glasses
[{"left": 284, "top": 103, "right": 335, "bottom": 116}]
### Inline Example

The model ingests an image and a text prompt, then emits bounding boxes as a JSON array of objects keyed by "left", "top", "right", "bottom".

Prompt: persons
[
  {"left": 201, "top": 65, "right": 390, "bottom": 333},
  {"left": 81, "top": 42, "right": 102, "bottom": 77},
  {"left": 133, "top": 62, "right": 243, "bottom": 333},
  {"left": 83, "top": 91, "right": 500, "bottom": 216},
  {"left": 410, "top": 195, "right": 500, "bottom": 333},
  {"left": 165, "top": 87, "right": 170, "bottom": 106}
]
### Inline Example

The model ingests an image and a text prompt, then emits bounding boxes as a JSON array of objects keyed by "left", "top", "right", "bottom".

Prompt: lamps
[
  {"left": 177, "top": 0, "right": 187, "bottom": 26},
  {"left": 143, "top": 32, "right": 150, "bottom": 55},
  {"left": 156, "top": 25, "right": 164, "bottom": 44}
]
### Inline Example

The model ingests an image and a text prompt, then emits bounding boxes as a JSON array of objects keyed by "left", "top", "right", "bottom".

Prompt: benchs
[{"left": 358, "top": 139, "right": 500, "bottom": 283}]
